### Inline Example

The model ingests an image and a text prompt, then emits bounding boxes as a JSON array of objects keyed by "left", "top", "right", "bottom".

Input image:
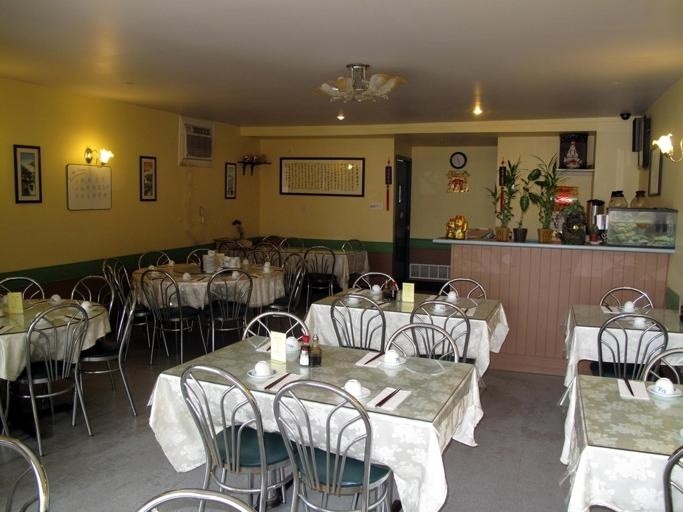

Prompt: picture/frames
[
  {"left": 13, "top": 144, "right": 42, "bottom": 203},
  {"left": 225, "top": 162, "right": 237, "bottom": 198},
  {"left": 279, "top": 157, "right": 365, "bottom": 197},
  {"left": 649, "top": 147, "right": 662, "bottom": 196},
  {"left": 139, "top": 155, "right": 157, "bottom": 201}
]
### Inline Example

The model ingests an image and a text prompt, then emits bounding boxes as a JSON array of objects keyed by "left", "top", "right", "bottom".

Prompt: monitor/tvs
[{"left": 639, "top": 115, "right": 649, "bottom": 169}]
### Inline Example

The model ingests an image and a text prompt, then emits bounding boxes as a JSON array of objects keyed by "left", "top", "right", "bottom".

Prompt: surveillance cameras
[{"left": 620, "top": 113, "right": 630, "bottom": 120}]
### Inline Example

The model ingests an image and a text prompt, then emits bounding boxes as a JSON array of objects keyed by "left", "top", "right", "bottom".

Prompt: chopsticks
[
  {"left": 605, "top": 304, "right": 613, "bottom": 312},
  {"left": 623, "top": 375, "right": 634, "bottom": 397},
  {"left": 0, "top": 260, "right": 283, "bottom": 329},
  {"left": 375, "top": 387, "right": 401, "bottom": 407},
  {"left": 265, "top": 373, "right": 291, "bottom": 389},
  {"left": 364, "top": 351, "right": 386, "bottom": 365},
  {"left": 355, "top": 285, "right": 468, "bottom": 315}
]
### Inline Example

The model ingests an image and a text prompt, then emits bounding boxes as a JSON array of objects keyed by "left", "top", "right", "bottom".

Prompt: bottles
[
  {"left": 299, "top": 335, "right": 311, "bottom": 368},
  {"left": 310, "top": 336, "right": 321, "bottom": 368}
]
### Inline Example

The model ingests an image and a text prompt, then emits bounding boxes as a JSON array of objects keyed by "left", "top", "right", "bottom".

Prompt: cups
[
  {"left": 286, "top": 336, "right": 298, "bottom": 349},
  {"left": 623, "top": 301, "right": 634, "bottom": 312},
  {"left": 252, "top": 361, "right": 272, "bottom": 377},
  {"left": 344, "top": 380, "right": 361, "bottom": 397},
  {"left": 654, "top": 378, "right": 674, "bottom": 393},
  {"left": 633, "top": 317, "right": 645, "bottom": 329},
  {"left": 384, "top": 350, "right": 400, "bottom": 365}
]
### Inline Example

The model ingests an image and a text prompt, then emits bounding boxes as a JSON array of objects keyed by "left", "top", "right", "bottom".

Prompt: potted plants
[
  {"left": 485, "top": 154, "right": 527, "bottom": 241},
  {"left": 513, "top": 168, "right": 547, "bottom": 243},
  {"left": 526, "top": 152, "right": 571, "bottom": 243}
]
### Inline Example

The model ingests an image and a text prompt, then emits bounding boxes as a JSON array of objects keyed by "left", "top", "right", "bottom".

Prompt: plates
[
  {"left": 647, "top": 384, "right": 681, "bottom": 397},
  {"left": 247, "top": 369, "right": 276, "bottom": 379},
  {"left": 378, "top": 357, "right": 406, "bottom": 366},
  {"left": 286, "top": 347, "right": 301, "bottom": 351},
  {"left": 337, "top": 387, "right": 371, "bottom": 399}
]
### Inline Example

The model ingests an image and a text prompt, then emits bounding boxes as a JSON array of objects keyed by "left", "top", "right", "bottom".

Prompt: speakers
[{"left": 632, "top": 118, "right": 640, "bottom": 152}]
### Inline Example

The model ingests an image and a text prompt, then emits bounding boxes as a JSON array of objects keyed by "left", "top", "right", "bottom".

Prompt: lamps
[
  {"left": 652, "top": 133, "right": 682, "bottom": 163},
  {"left": 93, "top": 148, "right": 114, "bottom": 167},
  {"left": 312, "top": 62, "right": 409, "bottom": 104}
]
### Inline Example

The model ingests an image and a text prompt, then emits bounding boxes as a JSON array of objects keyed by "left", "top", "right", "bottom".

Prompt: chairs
[
  {"left": 644, "top": 346, "right": 683, "bottom": 383},
  {"left": 597, "top": 312, "right": 668, "bottom": 380},
  {"left": 662, "top": 446, "right": 683, "bottom": 511},
  {"left": 598, "top": 287, "right": 655, "bottom": 307}
]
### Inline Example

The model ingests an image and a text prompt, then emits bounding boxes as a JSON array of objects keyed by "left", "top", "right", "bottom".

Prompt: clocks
[{"left": 450, "top": 151, "right": 467, "bottom": 169}]
